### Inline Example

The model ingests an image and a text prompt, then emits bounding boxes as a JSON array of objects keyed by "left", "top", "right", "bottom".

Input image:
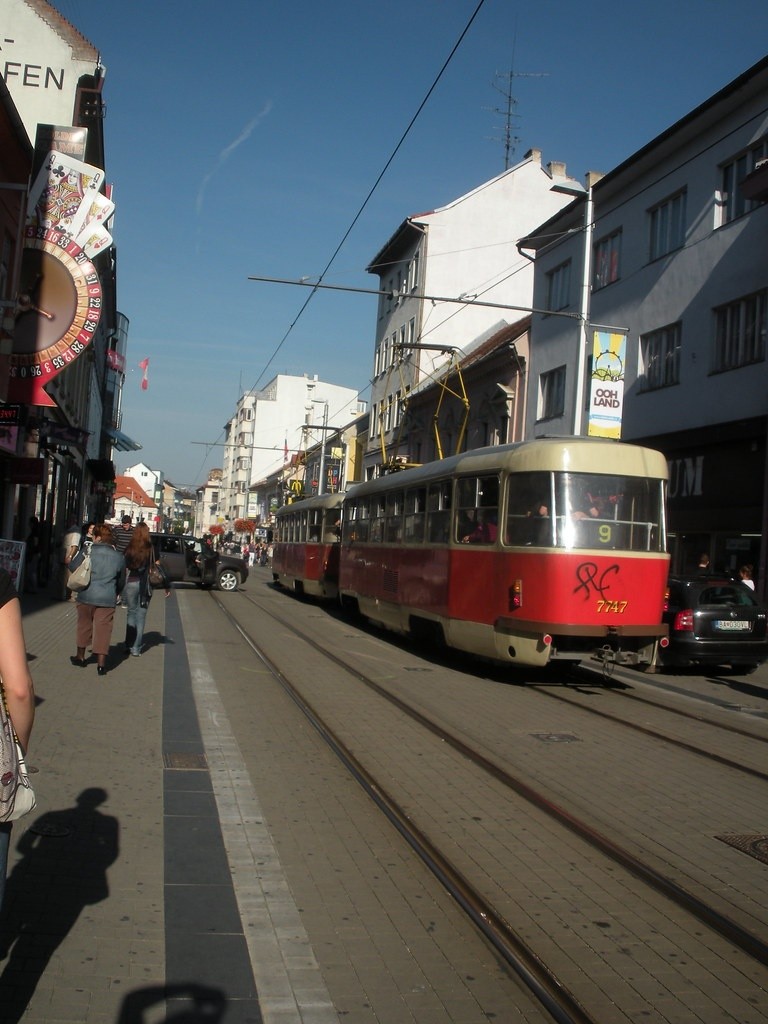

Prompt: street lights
[
  {"left": 127, "top": 486, "right": 142, "bottom": 521},
  {"left": 312, "top": 397, "right": 329, "bottom": 496}
]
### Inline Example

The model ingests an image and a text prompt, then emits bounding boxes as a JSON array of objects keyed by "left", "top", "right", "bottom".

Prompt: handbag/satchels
[
  {"left": 0, "top": 695, "right": 36, "bottom": 822},
  {"left": 149, "top": 546, "right": 168, "bottom": 590},
  {"left": 67, "top": 543, "right": 92, "bottom": 592}
]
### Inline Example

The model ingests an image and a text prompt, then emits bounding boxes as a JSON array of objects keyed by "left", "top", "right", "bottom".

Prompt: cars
[{"left": 150, "top": 532, "right": 249, "bottom": 593}]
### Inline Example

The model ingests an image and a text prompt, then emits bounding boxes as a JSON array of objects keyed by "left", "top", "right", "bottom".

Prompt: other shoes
[
  {"left": 117, "top": 642, "right": 130, "bottom": 653},
  {"left": 98, "top": 664, "right": 105, "bottom": 675},
  {"left": 70, "top": 655, "right": 87, "bottom": 667},
  {"left": 129, "top": 647, "right": 140, "bottom": 656}
]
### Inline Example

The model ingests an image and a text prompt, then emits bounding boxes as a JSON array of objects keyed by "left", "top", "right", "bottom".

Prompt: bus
[{"left": 271, "top": 433, "right": 674, "bottom": 668}]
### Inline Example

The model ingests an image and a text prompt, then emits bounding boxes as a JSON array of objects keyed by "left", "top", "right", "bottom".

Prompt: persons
[
  {"left": 740, "top": 564, "right": 755, "bottom": 591},
  {"left": 692, "top": 552, "right": 711, "bottom": 575},
  {"left": 220, "top": 540, "right": 274, "bottom": 569},
  {"left": 462, "top": 503, "right": 510, "bottom": 544},
  {"left": 0, "top": 567, "right": 36, "bottom": 905},
  {"left": 57, "top": 510, "right": 170, "bottom": 676},
  {"left": 528, "top": 491, "right": 599, "bottom": 547}
]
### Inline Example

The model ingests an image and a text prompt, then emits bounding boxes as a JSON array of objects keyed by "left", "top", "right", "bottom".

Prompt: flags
[{"left": 139, "top": 358, "right": 148, "bottom": 390}]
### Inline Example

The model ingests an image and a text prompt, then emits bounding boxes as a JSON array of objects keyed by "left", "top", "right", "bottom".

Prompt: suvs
[{"left": 665, "top": 573, "right": 768, "bottom": 675}]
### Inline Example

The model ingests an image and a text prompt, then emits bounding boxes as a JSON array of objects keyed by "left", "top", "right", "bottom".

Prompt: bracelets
[{"left": 69, "top": 555, "right": 73, "bottom": 558}]
